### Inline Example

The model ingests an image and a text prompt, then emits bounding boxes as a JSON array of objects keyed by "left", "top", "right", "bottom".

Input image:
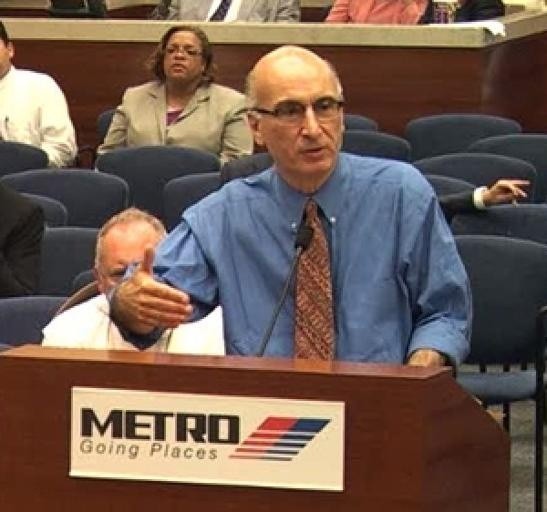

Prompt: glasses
[{"left": 248, "top": 94, "right": 344, "bottom": 125}]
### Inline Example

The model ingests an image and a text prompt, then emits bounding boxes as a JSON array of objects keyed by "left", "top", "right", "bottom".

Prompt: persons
[
  {"left": 220, "top": 152, "right": 532, "bottom": 226},
  {"left": 166, "top": 1, "right": 303, "bottom": 22},
  {"left": 323, "top": 0, "right": 430, "bottom": 24},
  {"left": 0, "top": 182, "right": 45, "bottom": 298},
  {"left": 94, "top": 24, "right": 254, "bottom": 171},
  {"left": 41, "top": 206, "right": 226, "bottom": 357},
  {"left": 417, "top": 1, "right": 505, "bottom": 24},
  {"left": 105, "top": 43, "right": 474, "bottom": 382},
  {"left": 0, "top": 20, "right": 79, "bottom": 169}
]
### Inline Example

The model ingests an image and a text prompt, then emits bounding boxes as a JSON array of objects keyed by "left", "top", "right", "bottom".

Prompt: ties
[
  {"left": 210, "top": 0, "right": 232, "bottom": 21},
  {"left": 294, "top": 200, "right": 335, "bottom": 359}
]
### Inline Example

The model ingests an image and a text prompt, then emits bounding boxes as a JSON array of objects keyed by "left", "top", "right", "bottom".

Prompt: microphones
[{"left": 255, "top": 223, "right": 315, "bottom": 357}]
